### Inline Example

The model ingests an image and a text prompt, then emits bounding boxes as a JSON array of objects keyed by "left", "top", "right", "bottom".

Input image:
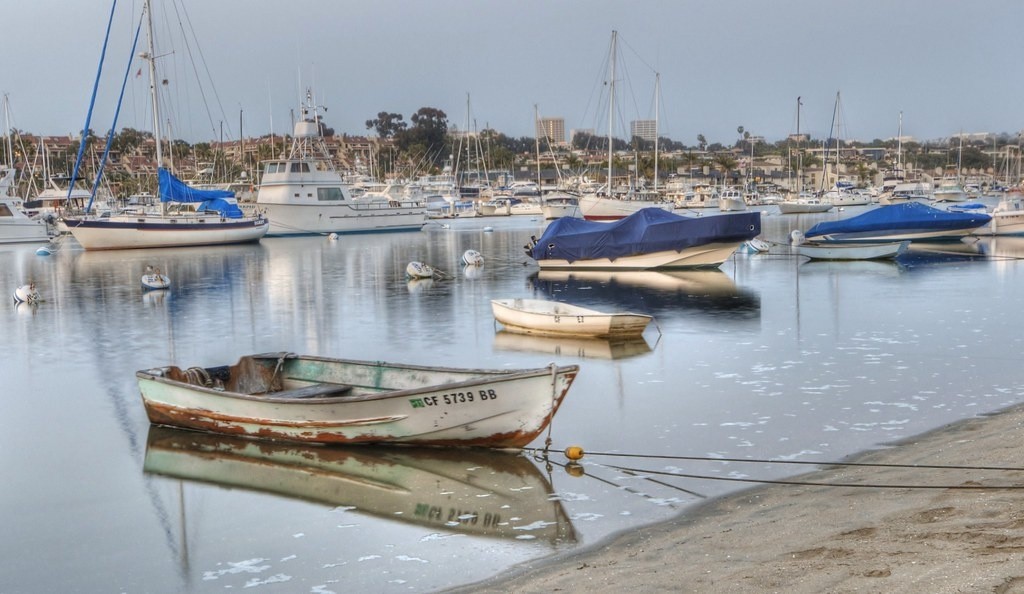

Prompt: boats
[
  {"left": 525, "top": 203, "right": 761, "bottom": 271},
  {"left": 491, "top": 297, "right": 653, "bottom": 340},
  {"left": 142, "top": 423, "right": 582, "bottom": 550},
  {"left": 537, "top": 267, "right": 735, "bottom": 297},
  {"left": 136, "top": 352, "right": 579, "bottom": 451},
  {"left": 494, "top": 331, "right": 652, "bottom": 361}
]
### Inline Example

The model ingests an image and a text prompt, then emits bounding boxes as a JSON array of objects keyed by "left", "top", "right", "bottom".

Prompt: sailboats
[{"left": 0, "top": 0, "right": 1024, "bottom": 249}]
